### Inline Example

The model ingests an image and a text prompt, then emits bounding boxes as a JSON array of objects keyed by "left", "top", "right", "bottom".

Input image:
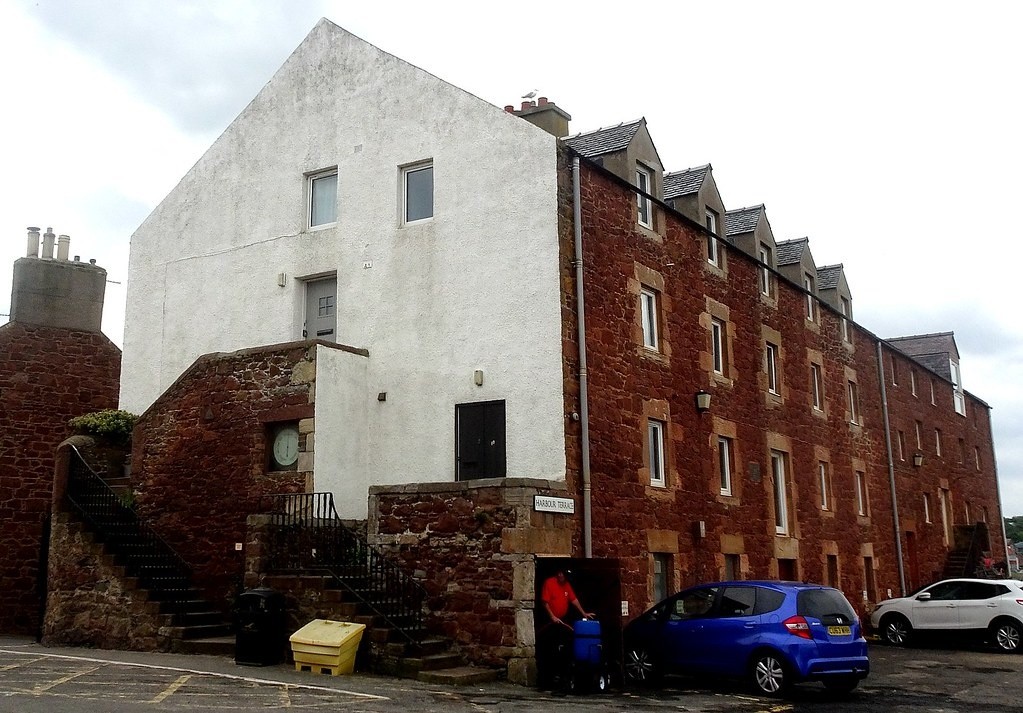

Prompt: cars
[
  {"left": 623, "top": 580, "right": 870, "bottom": 699},
  {"left": 871, "top": 578, "right": 1023, "bottom": 655}
]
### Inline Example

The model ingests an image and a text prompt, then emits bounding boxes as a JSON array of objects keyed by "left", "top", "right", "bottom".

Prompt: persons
[{"left": 541, "top": 564, "right": 596, "bottom": 689}]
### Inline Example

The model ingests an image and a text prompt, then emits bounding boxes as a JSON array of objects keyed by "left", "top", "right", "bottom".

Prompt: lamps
[
  {"left": 913, "top": 453, "right": 924, "bottom": 467},
  {"left": 694, "top": 391, "right": 712, "bottom": 414}
]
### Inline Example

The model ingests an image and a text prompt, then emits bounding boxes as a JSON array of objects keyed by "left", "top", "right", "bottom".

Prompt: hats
[{"left": 561, "top": 566, "right": 575, "bottom": 579}]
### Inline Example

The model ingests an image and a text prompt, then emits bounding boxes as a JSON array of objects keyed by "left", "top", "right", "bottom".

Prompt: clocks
[{"left": 273, "top": 428, "right": 299, "bottom": 466}]
